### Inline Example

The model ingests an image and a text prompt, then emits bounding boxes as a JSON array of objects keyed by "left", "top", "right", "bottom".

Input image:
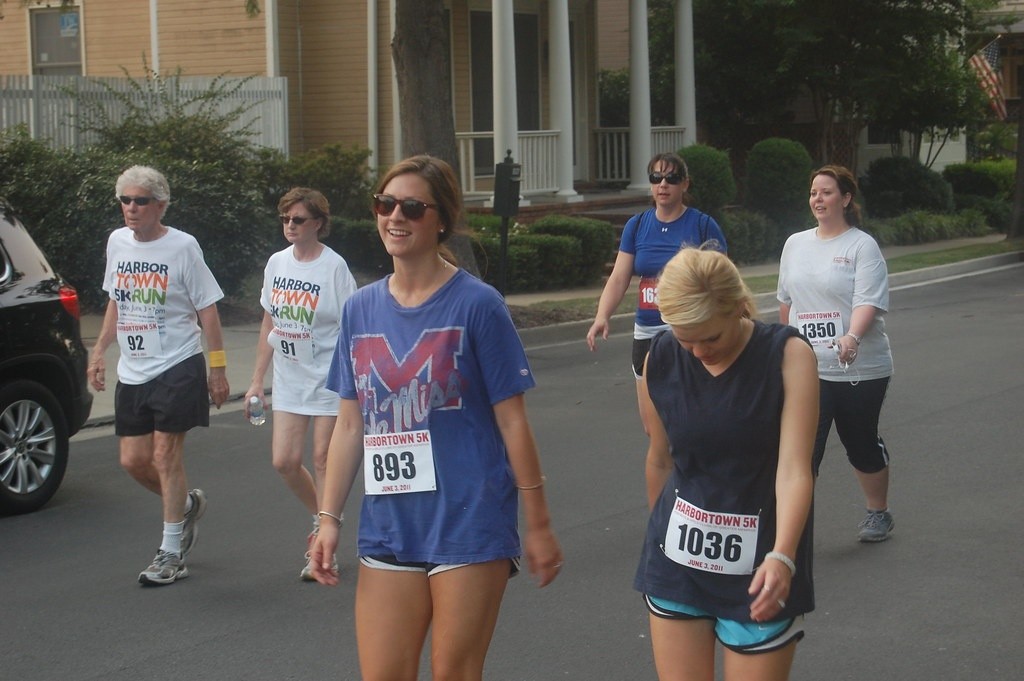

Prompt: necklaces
[{"left": 390, "top": 259, "right": 447, "bottom": 307}]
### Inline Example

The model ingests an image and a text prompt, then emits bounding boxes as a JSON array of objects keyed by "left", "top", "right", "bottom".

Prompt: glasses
[
  {"left": 373, "top": 193, "right": 443, "bottom": 220},
  {"left": 279, "top": 215, "right": 310, "bottom": 225},
  {"left": 648, "top": 171, "right": 685, "bottom": 185},
  {"left": 119, "top": 195, "right": 154, "bottom": 205}
]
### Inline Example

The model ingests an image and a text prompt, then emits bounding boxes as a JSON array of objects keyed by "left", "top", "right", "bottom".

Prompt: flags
[{"left": 968, "top": 36, "right": 1007, "bottom": 121}]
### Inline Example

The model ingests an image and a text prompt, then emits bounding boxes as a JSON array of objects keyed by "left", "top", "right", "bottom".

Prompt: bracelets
[
  {"left": 516, "top": 477, "right": 545, "bottom": 489},
  {"left": 318, "top": 512, "right": 340, "bottom": 524},
  {"left": 208, "top": 351, "right": 227, "bottom": 368},
  {"left": 765, "top": 550, "right": 796, "bottom": 576}
]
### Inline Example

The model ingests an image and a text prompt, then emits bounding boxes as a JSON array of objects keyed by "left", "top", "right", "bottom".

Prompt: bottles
[{"left": 248, "top": 396, "right": 265, "bottom": 426}]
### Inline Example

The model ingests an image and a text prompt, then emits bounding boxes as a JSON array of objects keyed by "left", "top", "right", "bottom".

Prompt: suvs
[{"left": 0, "top": 194, "right": 93, "bottom": 513}]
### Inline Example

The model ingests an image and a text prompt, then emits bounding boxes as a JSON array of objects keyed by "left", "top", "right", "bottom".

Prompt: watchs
[{"left": 847, "top": 332, "right": 861, "bottom": 344}]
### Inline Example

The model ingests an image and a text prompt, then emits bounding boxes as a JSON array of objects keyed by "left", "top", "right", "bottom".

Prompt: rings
[
  {"left": 777, "top": 599, "right": 785, "bottom": 608},
  {"left": 764, "top": 585, "right": 773, "bottom": 594},
  {"left": 554, "top": 565, "right": 560, "bottom": 568}
]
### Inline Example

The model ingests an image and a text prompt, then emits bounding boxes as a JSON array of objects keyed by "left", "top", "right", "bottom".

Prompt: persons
[
  {"left": 776, "top": 165, "right": 895, "bottom": 542},
  {"left": 632, "top": 247, "right": 820, "bottom": 681},
  {"left": 586, "top": 152, "right": 727, "bottom": 437},
  {"left": 244, "top": 187, "right": 358, "bottom": 582},
  {"left": 87, "top": 164, "right": 230, "bottom": 585},
  {"left": 310, "top": 154, "right": 563, "bottom": 681}
]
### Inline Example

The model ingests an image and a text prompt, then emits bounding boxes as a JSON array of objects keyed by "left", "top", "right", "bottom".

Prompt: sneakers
[
  {"left": 301, "top": 550, "right": 340, "bottom": 582},
  {"left": 858, "top": 510, "right": 896, "bottom": 542},
  {"left": 179, "top": 488, "right": 207, "bottom": 555},
  {"left": 138, "top": 548, "right": 189, "bottom": 584},
  {"left": 308, "top": 512, "right": 346, "bottom": 551}
]
